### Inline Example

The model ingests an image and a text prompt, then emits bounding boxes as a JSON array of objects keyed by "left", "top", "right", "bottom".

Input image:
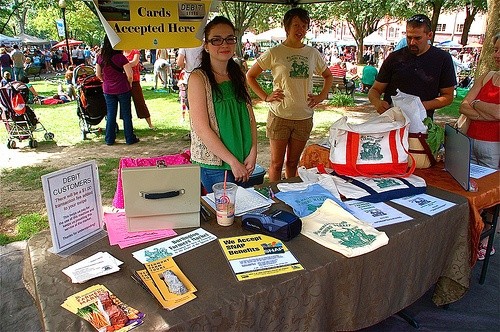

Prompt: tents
[
  {"left": 241, "top": 28, "right": 392, "bottom": 47},
  {"left": 0, "top": 30, "right": 57, "bottom": 51}
]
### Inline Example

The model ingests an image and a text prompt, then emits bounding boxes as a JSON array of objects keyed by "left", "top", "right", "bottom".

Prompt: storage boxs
[{"left": 121, "top": 160, "right": 200, "bottom": 232}]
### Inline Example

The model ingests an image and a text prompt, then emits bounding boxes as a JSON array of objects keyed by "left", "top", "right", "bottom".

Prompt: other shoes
[
  {"left": 361, "top": 89, "right": 365, "bottom": 93},
  {"left": 332, "top": 91, "right": 336, "bottom": 94},
  {"left": 106, "top": 141, "right": 115, "bottom": 145},
  {"left": 148, "top": 125, "right": 153, "bottom": 130},
  {"left": 128, "top": 137, "right": 139, "bottom": 145}
]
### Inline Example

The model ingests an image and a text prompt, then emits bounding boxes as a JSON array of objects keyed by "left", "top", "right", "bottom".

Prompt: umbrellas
[
  {"left": 52, "top": 40, "right": 82, "bottom": 56},
  {"left": 436, "top": 41, "right": 482, "bottom": 50}
]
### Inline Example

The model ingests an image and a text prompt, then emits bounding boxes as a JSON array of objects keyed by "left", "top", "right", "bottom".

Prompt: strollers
[
  {"left": 0, "top": 82, "right": 55, "bottom": 149},
  {"left": 72, "top": 64, "right": 119, "bottom": 140}
]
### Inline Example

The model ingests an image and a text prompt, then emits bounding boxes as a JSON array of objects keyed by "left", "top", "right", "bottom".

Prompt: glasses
[
  {"left": 206, "top": 34, "right": 238, "bottom": 46},
  {"left": 406, "top": 16, "right": 432, "bottom": 31}
]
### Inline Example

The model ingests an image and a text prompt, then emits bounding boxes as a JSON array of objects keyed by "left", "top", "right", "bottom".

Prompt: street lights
[{"left": 58, "top": 0, "right": 72, "bottom": 66}]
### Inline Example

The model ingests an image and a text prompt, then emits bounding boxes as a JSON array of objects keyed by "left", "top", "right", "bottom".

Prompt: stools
[{"left": 362, "top": 83, "right": 372, "bottom": 93}]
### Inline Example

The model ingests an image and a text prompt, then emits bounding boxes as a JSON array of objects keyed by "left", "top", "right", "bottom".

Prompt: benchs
[
  {"left": 256, "top": 72, "right": 355, "bottom": 98},
  {"left": 24, "top": 65, "right": 43, "bottom": 81}
]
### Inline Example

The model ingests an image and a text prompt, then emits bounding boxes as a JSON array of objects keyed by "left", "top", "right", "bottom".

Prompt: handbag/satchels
[
  {"left": 329, "top": 106, "right": 416, "bottom": 179},
  {"left": 408, "top": 132, "right": 436, "bottom": 169},
  {"left": 452, "top": 71, "right": 496, "bottom": 135},
  {"left": 390, "top": 88, "right": 428, "bottom": 134}
]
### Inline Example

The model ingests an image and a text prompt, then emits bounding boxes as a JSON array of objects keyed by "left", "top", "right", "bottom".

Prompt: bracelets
[
  {"left": 477, "top": 113, "right": 480, "bottom": 120},
  {"left": 264, "top": 93, "right": 269, "bottom": 102}
]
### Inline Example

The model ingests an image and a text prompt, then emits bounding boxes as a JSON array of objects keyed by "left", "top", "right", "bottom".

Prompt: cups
[{"left": 212, "top": 182, "right": 237, "bottom": 227}]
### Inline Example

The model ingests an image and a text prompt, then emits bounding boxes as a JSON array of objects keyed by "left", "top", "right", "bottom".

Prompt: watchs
[{"left": 472, "top": 100, "right": 480, "bottom": 110}]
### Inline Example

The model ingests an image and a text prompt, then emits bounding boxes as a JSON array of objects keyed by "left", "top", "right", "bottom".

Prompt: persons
[
  {"left": 0, "top": 34, "right": 398, "bottom": 145},
  {"left": 188, "top": 16, "right": 257, "bottom": 193},
  {"left": 448, "top": 47, "right": 482, "bottom": 96},
  {"left": 369, "top": 14, "right": 458, "bottom": 134},
  {"left": 247, "top": 7, "right": 335, "bottom": 181},
  {"left": 459, "top": 33, "right": 500, "bottom": 260}
]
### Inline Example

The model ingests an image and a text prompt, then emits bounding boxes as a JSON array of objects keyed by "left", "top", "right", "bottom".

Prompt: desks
[
  {"left": 21, "top": 167, "right": 471, "bottom": 332},
  {"left": 295, "top": 140, "right": 500, "bottom": 285}
]
